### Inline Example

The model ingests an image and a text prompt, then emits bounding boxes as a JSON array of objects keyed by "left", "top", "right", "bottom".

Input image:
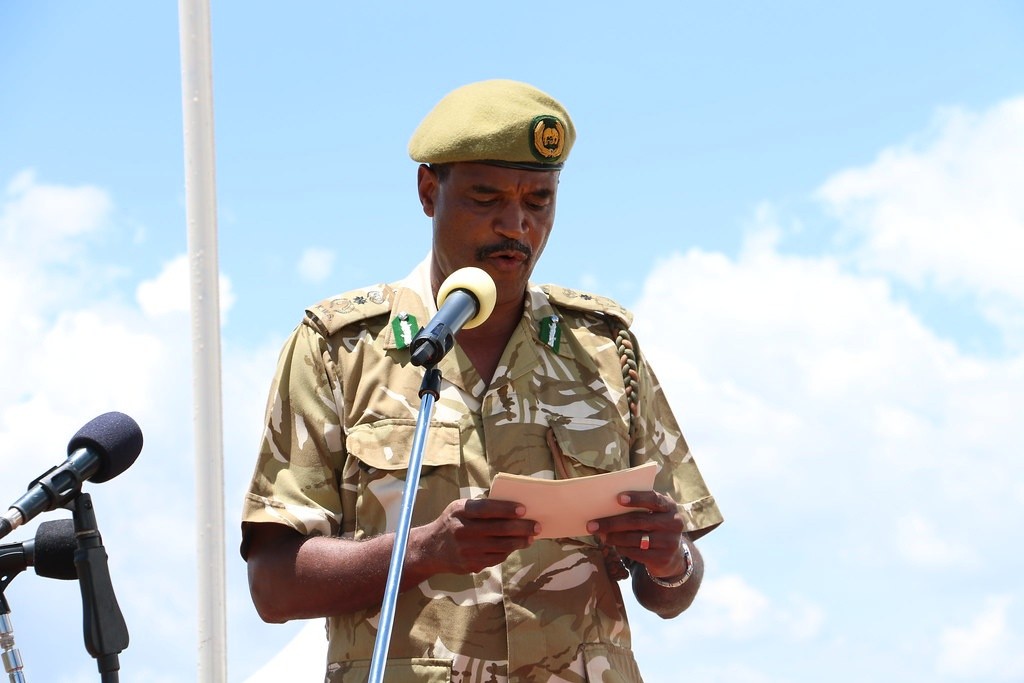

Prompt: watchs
[{"left": 645, "top": 540, "right": 694, "bottom": 588}]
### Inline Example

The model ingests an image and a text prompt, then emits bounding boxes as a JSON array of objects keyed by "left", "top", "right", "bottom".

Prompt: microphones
[
  {"left": 0, "top": 411, "right": 143, "bottom": 539},
  {"left": 0, "top": 518, "right": 78, "bottom": 580},
  {"left": 410, "top": 265, "right": 497, "bottom": 368}
]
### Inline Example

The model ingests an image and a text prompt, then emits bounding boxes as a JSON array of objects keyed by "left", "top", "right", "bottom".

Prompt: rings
[{"left": 640, "top": 536, "right": 649, "bottom": 550}]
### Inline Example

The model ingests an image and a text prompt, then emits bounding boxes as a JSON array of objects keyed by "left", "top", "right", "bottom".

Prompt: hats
[{"left": 408, "top": 78, "right": 576, "bottom": 172}]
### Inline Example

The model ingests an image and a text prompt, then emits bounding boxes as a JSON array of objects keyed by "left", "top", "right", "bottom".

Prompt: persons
[{"left": 239, "top": 80, "right": 724, "bottom": 683}]
